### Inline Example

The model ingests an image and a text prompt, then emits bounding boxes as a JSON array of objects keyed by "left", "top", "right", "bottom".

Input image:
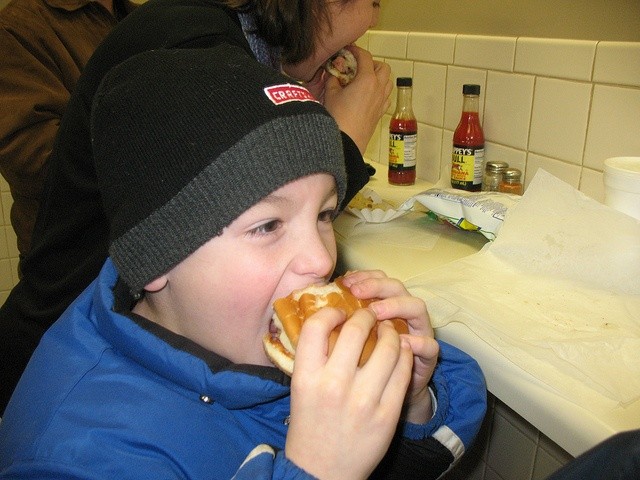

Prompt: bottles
[
  {"left": 499, "top": 168, "right": 522, "bottom": 194},
  {"left": 483, "top": 159, "right": 509, "bottom": 191},
  {"left": 388, "top": 75, "right": 419, "bottom": 187},
  {"left": 449, "top": 83, "right": 485, "bottom": 191}
]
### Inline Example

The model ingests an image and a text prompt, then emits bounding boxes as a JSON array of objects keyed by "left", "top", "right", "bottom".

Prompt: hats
[{"left": 90, "top": 46, "right": 348, "bottom": 294}]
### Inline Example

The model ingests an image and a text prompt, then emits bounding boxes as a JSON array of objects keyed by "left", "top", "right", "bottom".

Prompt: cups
[{"left": 604, "top": 156, "right": 640, "bottom": 220}]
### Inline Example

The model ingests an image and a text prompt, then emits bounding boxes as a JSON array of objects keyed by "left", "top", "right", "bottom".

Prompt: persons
[
  {"left": 0, "top": 44, "right": 489, "bottom": 480},
  {"left": 0, "top": 2, "right": 394, "bottom": 414},
  {"left": 0, "top": 0, "right": 143, "bottom": 280}
]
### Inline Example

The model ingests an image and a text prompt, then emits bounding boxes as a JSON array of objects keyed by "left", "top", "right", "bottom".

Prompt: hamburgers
[
  {"left": 263, "top": 277, "right": 409, "bottom": 377},
  {"left": 325, "top": 49, "right": 358, "bottom": 89}
]
